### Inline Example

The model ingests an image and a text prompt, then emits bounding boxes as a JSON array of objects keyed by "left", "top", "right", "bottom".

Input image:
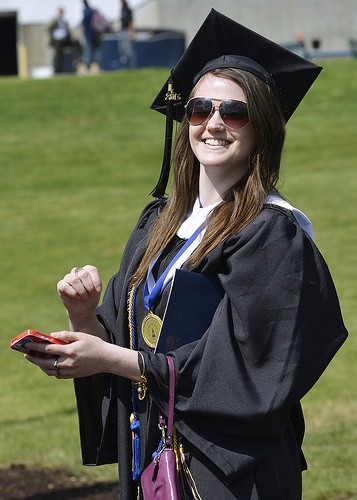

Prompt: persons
[
  {"left": 48, "top": 6, "right": 76, "bottom": 72},
  {"left": 117, "top": 0, "right": 139, "bottom": 69},
  {"left": 78, "top": 1, "right": 114, "bottom": 73},
  {"left": 21, "top": 53, "right": 324, "bottom": 499}
]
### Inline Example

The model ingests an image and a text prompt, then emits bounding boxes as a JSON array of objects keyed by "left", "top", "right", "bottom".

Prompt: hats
[{"left": 149, "top": 9, "right": 323, "bottom": 197}]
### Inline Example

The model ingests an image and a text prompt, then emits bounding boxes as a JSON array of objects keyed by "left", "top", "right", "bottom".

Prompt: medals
[{"left": 139, "top": 313, "right": 162, "bottom": 349}]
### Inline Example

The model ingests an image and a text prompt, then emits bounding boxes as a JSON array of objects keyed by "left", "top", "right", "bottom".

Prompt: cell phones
[{"left": 11, "top": 329, "right": 69, "bottom": 359}]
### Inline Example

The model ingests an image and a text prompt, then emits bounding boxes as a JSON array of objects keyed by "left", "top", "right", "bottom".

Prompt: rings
[
  {"left": 57, "top": 369, "right": 61, "bottom": 377},
  {"left": 54, "top": 357, "right": 61, "bottom": 370},
  {"left": 74, "top": 266, "right": 84, "bottom": 275}
]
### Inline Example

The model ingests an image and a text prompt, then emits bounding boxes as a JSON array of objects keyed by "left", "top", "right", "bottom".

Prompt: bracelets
[{"left": 130, "top": 346, "right": 148, "bottom": 402}]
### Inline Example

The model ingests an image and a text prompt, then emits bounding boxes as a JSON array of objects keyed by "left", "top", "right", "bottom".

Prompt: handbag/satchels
[{"left": 139, "top": 449, "right": 177, "bottom": 500}]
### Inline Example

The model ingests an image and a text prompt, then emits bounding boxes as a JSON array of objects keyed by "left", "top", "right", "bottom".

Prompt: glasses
[{"left": 183, "top": 95, "right": 250, "bottom": 130}]
